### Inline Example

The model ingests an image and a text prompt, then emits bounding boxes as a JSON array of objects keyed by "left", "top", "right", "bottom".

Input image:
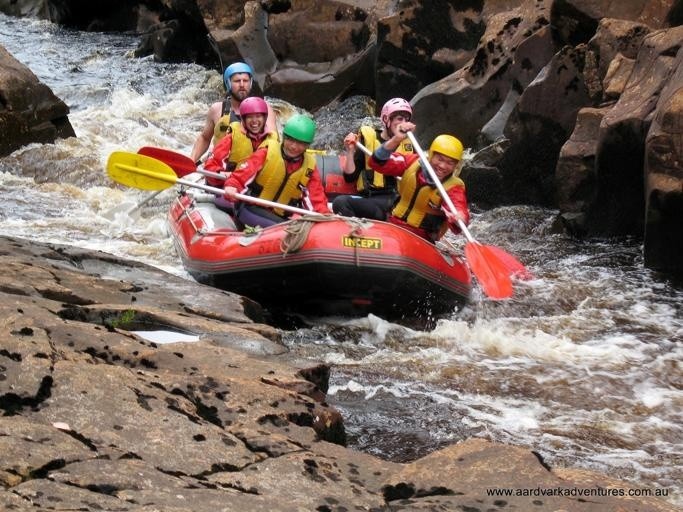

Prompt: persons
[
  {"left": 333, "top": 97, "right": 414, "bottom": 220},
  {"left": 203, "top": 96, "right": 279, "bottom": 211},
  {"left": 189, "top": 61, "right": 280, "bottom": 169},
  {"left": 367, "top": 120, "right": 471, "bottom": 245},
  {"left": 223, "top": 114, "right": 334, "bottom": 230}
]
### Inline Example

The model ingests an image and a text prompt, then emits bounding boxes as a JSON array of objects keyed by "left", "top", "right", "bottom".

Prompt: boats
[{"left": 169, "top": 151, "right": 470, "bottom": 314}]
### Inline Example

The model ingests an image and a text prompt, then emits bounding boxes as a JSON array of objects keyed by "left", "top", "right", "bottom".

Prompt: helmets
[
  {"left": 223, "top": 62, "right": 255, "bottom": 93},
  {"left": 381, "top": 98, "right": 412, "bottom": 125},
  {"left": 239, "top": 97, "right": 268, "bottom": 130},
  {"left": 283, "top": 113, "right": 316, "bottom": 144},
  {"left": 428, "top": 135, "right": 463, "bottom": 170}
]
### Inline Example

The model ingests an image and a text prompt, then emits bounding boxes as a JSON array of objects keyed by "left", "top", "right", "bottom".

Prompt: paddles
[
  {"left": 105, "top": 150, "right": 326, "bottom": 217},
  {"left": 137, "top": 146, "right": 233, "bottom": 179},
  {"left": 406, "top": 130, "right": 514, "bottom": 300},
  {"left": 353, "top": 140, "right": 535, "bottom": 281}
]
